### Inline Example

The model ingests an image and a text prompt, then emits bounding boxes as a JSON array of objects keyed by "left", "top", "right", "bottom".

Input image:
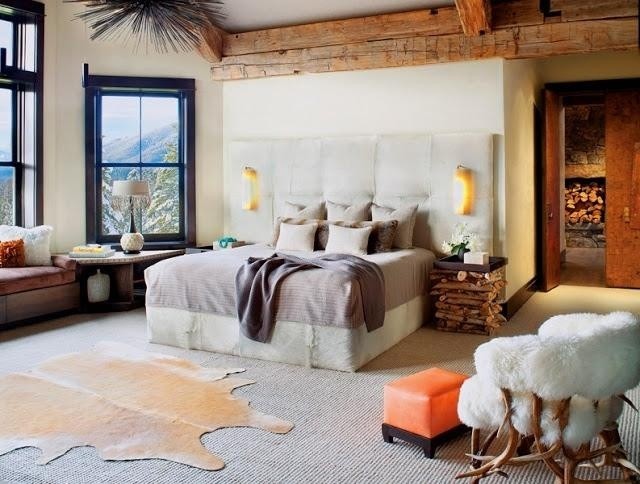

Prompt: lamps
[
  {"left": 242, "top": 167, "right": 259, "bottom": 210},
  {"left": 453, "top": 165, "right": 472, "bottom": 218},
  {"left": 112, "top": 180, "right": 149, "bottom": 255}
]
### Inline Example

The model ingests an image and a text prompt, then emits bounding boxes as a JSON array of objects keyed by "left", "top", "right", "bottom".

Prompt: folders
[{"left": 185, "top": 248, "right": 207, "bottom": 254}]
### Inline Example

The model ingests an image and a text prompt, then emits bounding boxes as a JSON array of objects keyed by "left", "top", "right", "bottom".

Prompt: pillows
[
  {"left": 354, "top": 219, "right": 398, "bottom": 251},
  {"left": 277, "top": 201, "right": 325, "bottom": 225},
  {"left": 371, "top": 202, "right": 419, "bottom": 249},
  {"left": 324, "top": 223, "right": 371, "bottom": 257},
  {"left": 324, "top": 200, "right": 371, "bottom": 231},
  {"left": 274, "top": 223, "right": 316, "bottom": 255},
  {"left": 315, "top": 220, "right": 377, "bottom": 250},
  {"left": 0, "top": 225, "right": 52, "bottom": 264},
  {"left": 0, "top": 239, "right": 24, "bottom": 267},
  {"left": 271, "top": 216, "right": 321, "bottom": 252}
]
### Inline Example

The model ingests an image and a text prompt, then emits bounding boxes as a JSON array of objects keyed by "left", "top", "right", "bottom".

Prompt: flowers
[{"left": 441, "top": 223, "right": 473, "bottom": 255}]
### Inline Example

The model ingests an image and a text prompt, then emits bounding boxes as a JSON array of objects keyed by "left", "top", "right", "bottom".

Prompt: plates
[{"left": 69, "top": 249, "right": 116, "bottom": 258}]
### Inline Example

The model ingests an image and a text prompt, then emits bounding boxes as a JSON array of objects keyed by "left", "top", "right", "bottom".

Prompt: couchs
[{"left": 0, "top": 255, "right": 81, "bottom": 331}]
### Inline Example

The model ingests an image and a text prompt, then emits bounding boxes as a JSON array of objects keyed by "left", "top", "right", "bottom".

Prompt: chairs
[{"left": 455, "top": 314, "right": 640, "bottom": 484}]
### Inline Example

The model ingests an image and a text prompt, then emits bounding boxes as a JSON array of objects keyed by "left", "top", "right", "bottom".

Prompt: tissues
[{"left": 464, "top": 244, "right": 489, "bottom": 265}]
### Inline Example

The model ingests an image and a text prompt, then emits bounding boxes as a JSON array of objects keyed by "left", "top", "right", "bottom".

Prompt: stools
[{"left": 380, "top": 367, "right": 473, "bottom": 459}]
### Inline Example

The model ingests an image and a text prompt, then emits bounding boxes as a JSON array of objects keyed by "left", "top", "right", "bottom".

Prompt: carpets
[{"left": 0, "top": 340, "right": 294, "bottom": 472}]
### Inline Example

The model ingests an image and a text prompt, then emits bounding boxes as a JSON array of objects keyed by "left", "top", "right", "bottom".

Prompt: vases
[{"left": 458, "top": 249, "right": 471, "bottom": 260}]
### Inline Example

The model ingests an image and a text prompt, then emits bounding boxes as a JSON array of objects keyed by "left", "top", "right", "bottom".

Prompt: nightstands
[{"left": 429, "top": 255, "right": 508, "bottom": 335}]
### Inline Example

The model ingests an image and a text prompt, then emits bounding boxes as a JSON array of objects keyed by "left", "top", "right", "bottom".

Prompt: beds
[{"left": 144, "top": 242, "right": 439, "bottom": 376}]
